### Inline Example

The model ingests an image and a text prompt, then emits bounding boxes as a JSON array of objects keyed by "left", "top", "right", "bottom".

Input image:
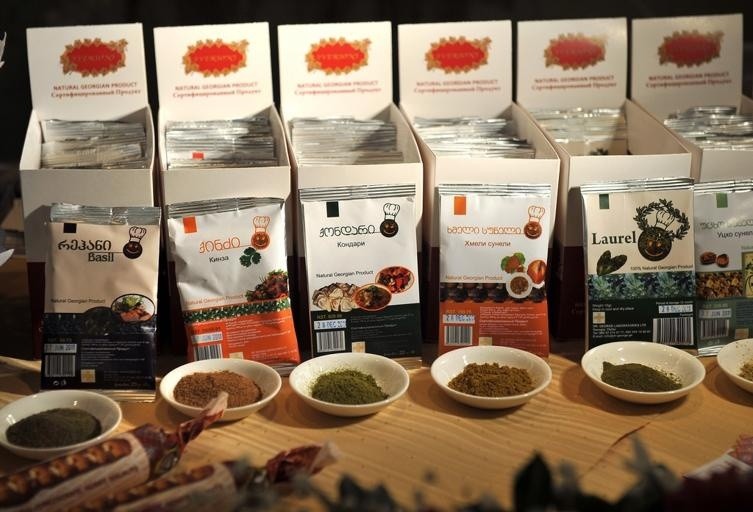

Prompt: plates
[
  {"left": 158, "top": 358, "right": 283, "bottom": 422},
  {"left": 355, "top": 266, "right": 415, "bottom": 312},
  {"left": 582, "top": 342, "right": 706, "bottom": 403},
  {"left": 289, "top": 353, "right": 410, "bottom": 417},
  {"left": 111, "top": 292, "right": 156, "bottom": 324},
  {"left": 504, "top": 273, "right": 533, "bottom": 300},
  {"left": 718, "top": 339, "right": 752, "bottom": 388},
  {"left": 431, "top": 347, "right": 553, "bottom": 409},
  {"left": 2, "top": 391, "right": 121, "bottom": 459}
]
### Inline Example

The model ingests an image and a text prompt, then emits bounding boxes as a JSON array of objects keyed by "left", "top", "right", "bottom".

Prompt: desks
[{"left": 0, "top": 255, "right": 753, "bottom": 512}]
